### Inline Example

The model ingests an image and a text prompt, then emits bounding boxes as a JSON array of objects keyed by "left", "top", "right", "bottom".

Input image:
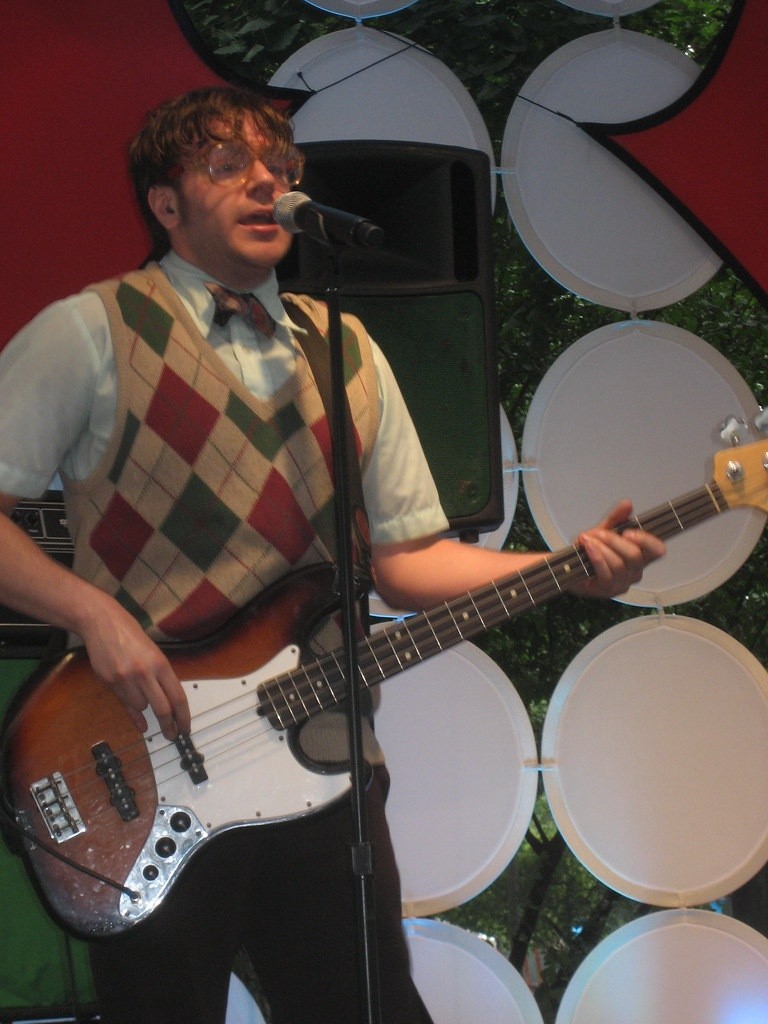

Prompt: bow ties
[{"left": 202, "top": 281, "right": 277, "bottom": 340}]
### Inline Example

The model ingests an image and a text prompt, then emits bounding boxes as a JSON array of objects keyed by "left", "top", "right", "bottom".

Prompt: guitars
[{"left": 1, "top": 409, "right": 767, "bottom": 941}]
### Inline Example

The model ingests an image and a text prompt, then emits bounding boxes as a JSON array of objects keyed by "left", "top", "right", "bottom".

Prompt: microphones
[{"left": 271, "top": 191, "right": 385, "bottom": 249}]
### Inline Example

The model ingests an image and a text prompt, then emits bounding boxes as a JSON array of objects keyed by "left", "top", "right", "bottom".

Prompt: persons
[{"left": 0, "top": 88, "right": 666, "bottom": 1024}]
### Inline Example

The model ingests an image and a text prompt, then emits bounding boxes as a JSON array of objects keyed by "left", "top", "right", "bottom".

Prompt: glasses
[{"left": 203, "top": 139, "right": 306, "bottom": 190}]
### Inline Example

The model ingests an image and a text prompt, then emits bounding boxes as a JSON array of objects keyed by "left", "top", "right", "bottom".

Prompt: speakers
[{"left": 277, "top": 140, "right": 506, "bottom": 545}]
